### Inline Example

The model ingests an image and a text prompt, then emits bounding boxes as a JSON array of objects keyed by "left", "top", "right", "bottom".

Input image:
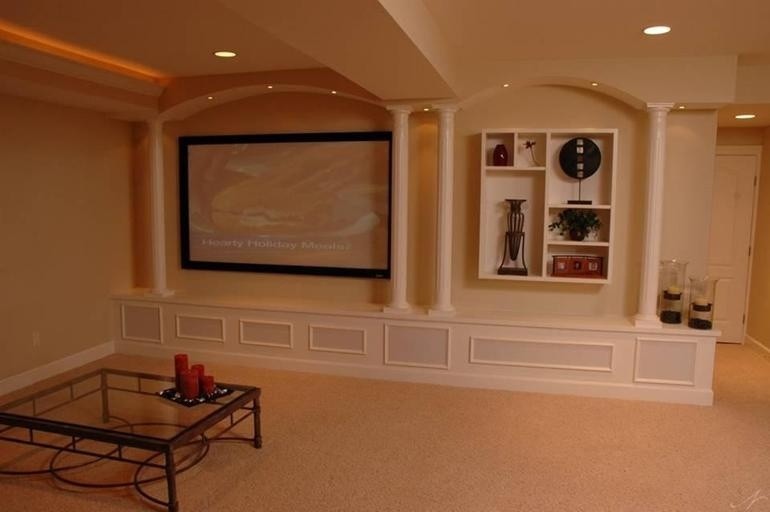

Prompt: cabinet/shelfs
[{"left": 478, "top": 126, "right": 619, "bottom": 284}]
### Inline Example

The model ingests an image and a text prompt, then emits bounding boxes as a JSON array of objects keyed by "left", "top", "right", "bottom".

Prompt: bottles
[
  {"left": 659, "top": 260, "right": 719, "bottom": 330},
  {"left": 493, "top": 144, "right": 508, "bottom": 165}
]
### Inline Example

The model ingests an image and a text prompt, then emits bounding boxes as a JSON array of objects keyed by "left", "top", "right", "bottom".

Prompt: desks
[{"left": 0, "top": 367, "right": 263, "bottom": 512}]
